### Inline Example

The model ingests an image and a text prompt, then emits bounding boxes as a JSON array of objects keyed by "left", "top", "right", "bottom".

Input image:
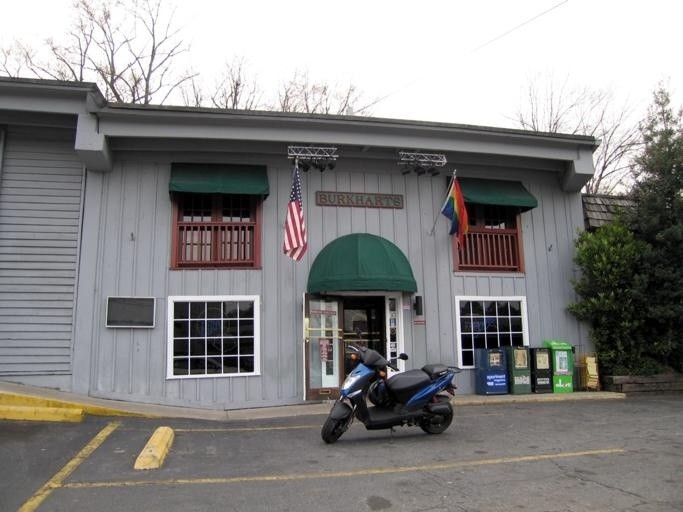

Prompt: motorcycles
[{"left": 321, "top": 328, "right": 462, "bottom": 445}]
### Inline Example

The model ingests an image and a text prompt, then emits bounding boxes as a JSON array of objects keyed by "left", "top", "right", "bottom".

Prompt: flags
[
  {"left": 441, "top": 176, "right": 468, "bottom": 238},
  {"left": 283, "top": 160, "right": 307, "bottom": 263}
]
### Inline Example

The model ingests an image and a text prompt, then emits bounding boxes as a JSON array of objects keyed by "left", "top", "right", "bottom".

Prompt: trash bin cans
[
  {"left": 505, "top": 345, "right": 532, "bottom": 395},
  {"left": 474, "top": 346, "right": 509, "bottom": 396},
  {"left": 530, "top": 347, "right": 553, "bottom": 395},
  {"left": 543, "top": 339, "right": 573, "bottom": 394}
]
